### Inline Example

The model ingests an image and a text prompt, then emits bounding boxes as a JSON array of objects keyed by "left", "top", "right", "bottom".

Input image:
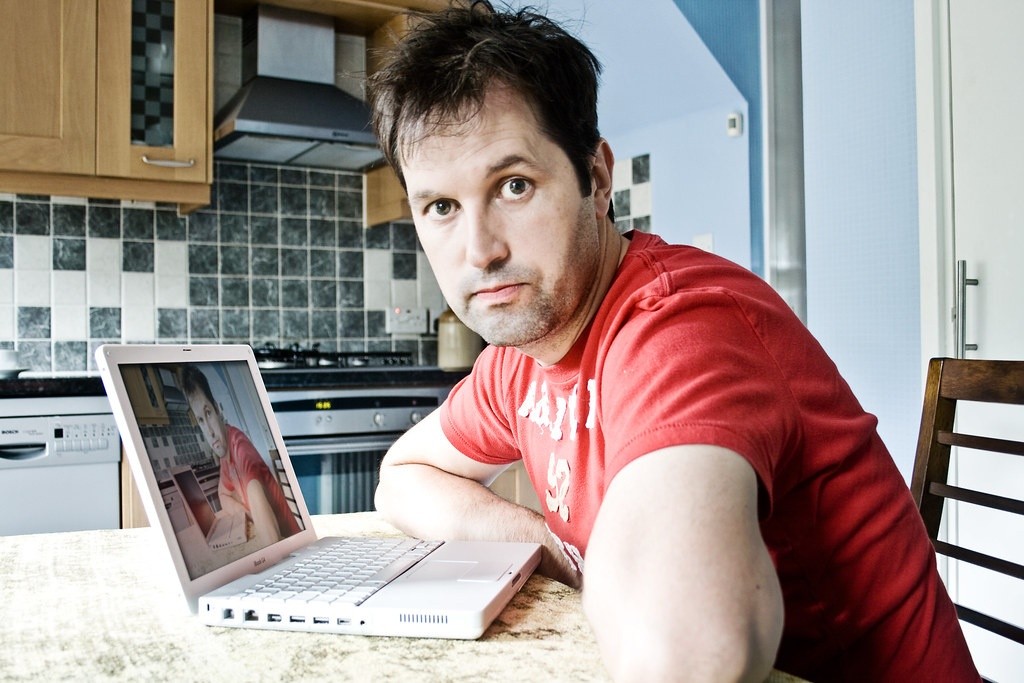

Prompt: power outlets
[
  {"left": 385, "top": 306, "right": 427, "bottom": 334},
  {"left": 429, "top": 310, "right": 447, "bottom": 334}
]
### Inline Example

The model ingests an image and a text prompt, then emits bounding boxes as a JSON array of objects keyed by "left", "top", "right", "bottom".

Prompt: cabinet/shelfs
[
  {"left": 1, "top": 0, "right": 216, "bottom": 216},
  {"left": 267, "top": 0, "right": 492, "bottom": 229},
  {"left": 119, "top": 363, "right": 170, "bottom": 425},
  {"left": 174, "top": 366, "right": 201, "bottom": 428}
]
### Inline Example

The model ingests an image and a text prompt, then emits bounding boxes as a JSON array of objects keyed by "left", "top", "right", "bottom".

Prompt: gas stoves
[{"left": 250, "top": 343, "right": 451, "bottom": 442}]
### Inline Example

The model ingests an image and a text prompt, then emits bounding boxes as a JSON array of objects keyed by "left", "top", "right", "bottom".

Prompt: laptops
[
  {"left": 95, "top": 343, "right": 542, "bottom": 641},
  {"left": 168, "top": 465, "right": 248, "bottom": 552}
]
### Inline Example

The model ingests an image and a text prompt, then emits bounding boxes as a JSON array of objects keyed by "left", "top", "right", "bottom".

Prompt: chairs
[
  {"left": 268, "top": 449, "right": 306, "bottom": 531},
  {"left": 911, "top": 358, "right": 1024, "bottom": 683}
]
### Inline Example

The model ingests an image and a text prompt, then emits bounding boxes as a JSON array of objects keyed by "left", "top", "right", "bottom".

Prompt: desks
[
  {"left": 0, "top": 510, "right": 811, "bottom": 683},
  {"left": 175, "top": 509, "right": 286, "bottom": 581}
]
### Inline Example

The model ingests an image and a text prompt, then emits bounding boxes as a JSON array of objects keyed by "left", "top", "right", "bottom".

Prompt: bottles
[{"left": 438, "top": 306, "right": 483, "bottom": 372}]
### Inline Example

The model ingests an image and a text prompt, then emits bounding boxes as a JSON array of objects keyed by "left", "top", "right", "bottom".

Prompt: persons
[
  {"left": 374, "top": 0, "right": 983, "bottom": 683},
  {"left": 180, "top": 364, "right": 302, "bottom": 546}
]
